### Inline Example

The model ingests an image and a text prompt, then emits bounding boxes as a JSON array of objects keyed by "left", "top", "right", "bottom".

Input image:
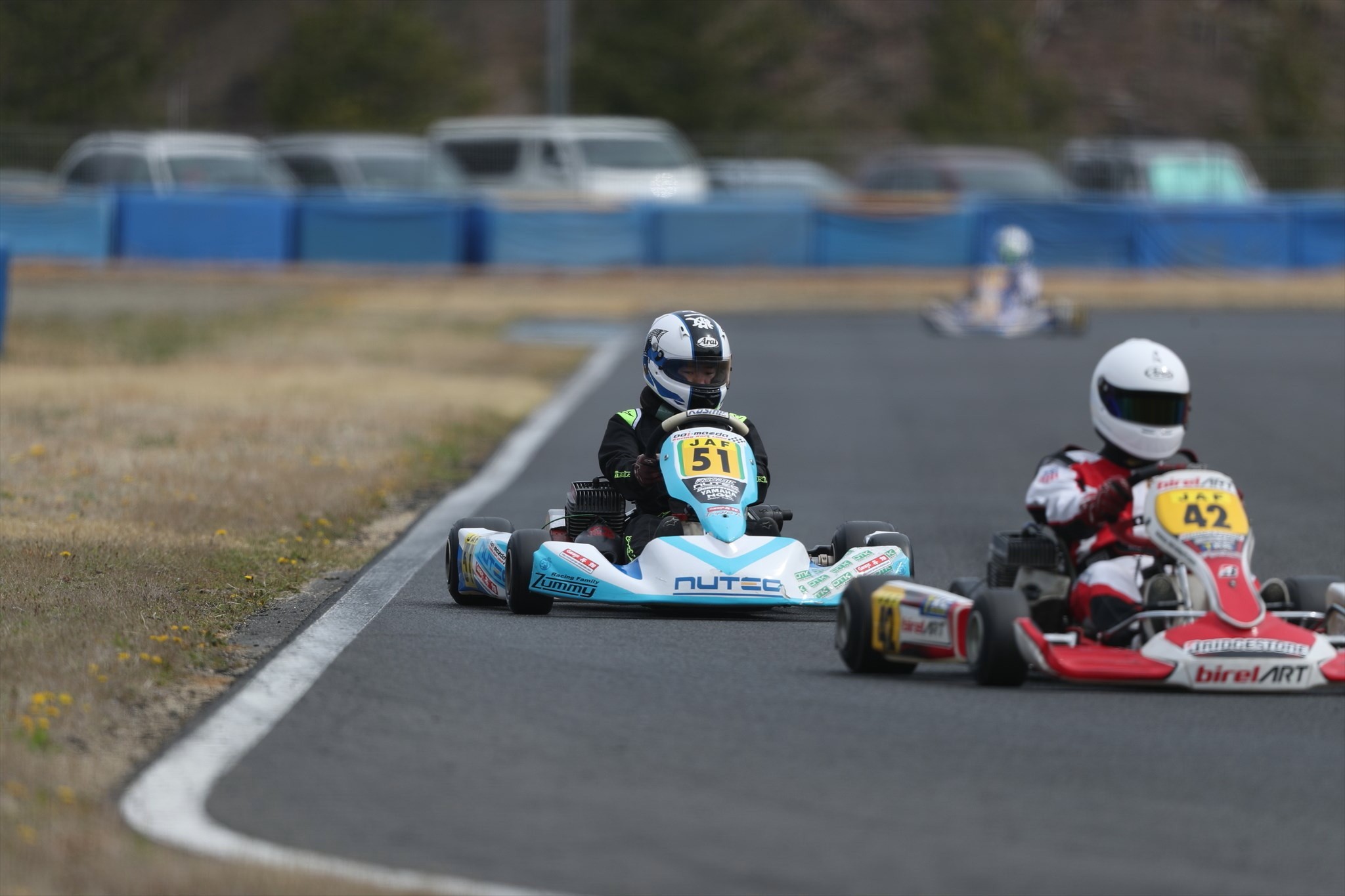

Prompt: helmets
[
  {"left": 1090, "top": 337, "right": 1191, "bottom": 462},
  {"left": 642, "top": 310, "right": 733, "bottom": 412},
  {"left": 997, "top": 227, "right": 1030, "bottom": 262}
]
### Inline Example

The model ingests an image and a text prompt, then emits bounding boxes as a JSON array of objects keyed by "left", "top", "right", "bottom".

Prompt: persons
[
  {"left": 957, "top": 225, "right": 1042, "bottom": 306},
  {"left": 1025, "top": 338, "right": 1302, "bottom": 646},
  {"left": 599, "top": 310, "right": 770, "bottom": 562}
]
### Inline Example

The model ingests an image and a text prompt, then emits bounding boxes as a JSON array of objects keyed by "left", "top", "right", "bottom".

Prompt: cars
[
  {"left": 267, "top": 129, "right": 464, "bottom": 196},
  {"left": 49, "top": 130, "right": 301, "bottom": 193},
  {"left": 704, "top": 132, "right": 1267, "bottom": 209}
]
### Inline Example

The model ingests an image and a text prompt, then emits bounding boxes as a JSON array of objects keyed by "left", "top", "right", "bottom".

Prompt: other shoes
[
  {"left": 652, "top": 516, "right": 684, "bottom": 538},
  {"left": 753, "top": 516, "right": 781, "bottom": 537},
  {"left": 1260, "top": 581, "right": 1294, "bottom": 611},
  {"left": 1139, "top": 573, "right": 1183, "bottom": 646}
]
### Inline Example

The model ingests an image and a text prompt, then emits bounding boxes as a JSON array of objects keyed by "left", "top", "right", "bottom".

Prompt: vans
[{"left": 424, "top": 117, "right": 710, "bottom": 204}]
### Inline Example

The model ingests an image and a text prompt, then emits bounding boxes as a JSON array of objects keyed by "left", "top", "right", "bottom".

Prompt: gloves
[
  {"left": 1080, "top": 474, "right": 1134, "bottom": 530},
  {"left": 634, "top": 453, "right": 664, "bottom": 484}
]
